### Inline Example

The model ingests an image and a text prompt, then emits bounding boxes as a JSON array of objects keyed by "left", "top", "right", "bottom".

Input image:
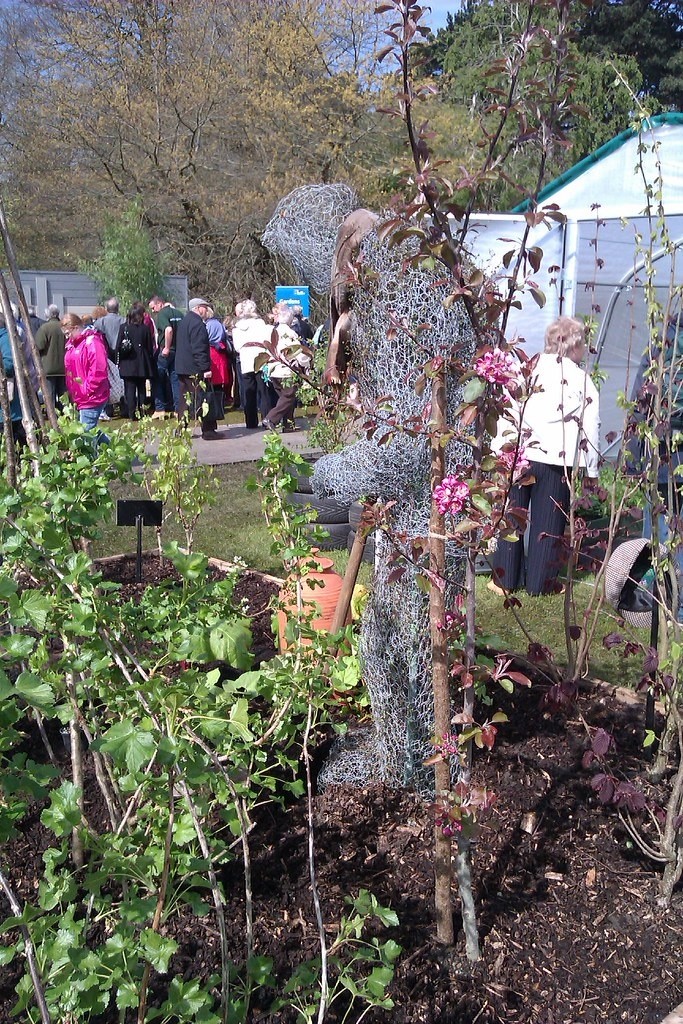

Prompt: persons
[
  {"left": 175, "top": 298, "right": 226, "bottom": 440},
  {"left": 623, "top": 314, "right": 683, "bottom": 621},
  {"left": 485, "top": 315, "right": 600, "bottom": 598},
  {"left": 57, "top": 313, "right": 115, "bottom": 477},
  {"left": 0, "top": 298, "right": 360, "bottom": 479}
]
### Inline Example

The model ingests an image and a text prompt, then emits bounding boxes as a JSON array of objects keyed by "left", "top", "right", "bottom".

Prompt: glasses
[{"left": 64, "top": 328, "right": 74, "bottom": 336}]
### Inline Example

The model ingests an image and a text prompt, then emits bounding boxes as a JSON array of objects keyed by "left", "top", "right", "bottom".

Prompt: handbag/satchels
[
  {"left": 121, "top": 323, "right": 136, "bottom": 359},
  {"left": 197, "top": 381, "right": 225, "bottom": 420}
]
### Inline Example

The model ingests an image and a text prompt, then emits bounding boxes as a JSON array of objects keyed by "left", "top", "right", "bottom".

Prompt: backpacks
[{"left": 86, "top": 337, "right": 124, "bottom": 404}]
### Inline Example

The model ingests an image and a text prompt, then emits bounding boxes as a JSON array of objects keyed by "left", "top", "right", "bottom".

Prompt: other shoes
[
  {"left": 488, "top": 580, "right": 509, "bottom": 595},
  {"left": 201, "top": 430, "right": 224, "bottom": 441},
  {"left": 282, "top": 427, "right": 296, "bottom": 433},
  {"left": 263, "top": 417, "right": 276, "bottom": 434},
  {"left": 560, "top": 584, "right": 566, "bottom": 593}
]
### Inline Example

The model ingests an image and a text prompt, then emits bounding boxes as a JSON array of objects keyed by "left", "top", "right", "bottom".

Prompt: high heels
[{"left": 151, "top": 411, "right": 166, "bottom": 420}]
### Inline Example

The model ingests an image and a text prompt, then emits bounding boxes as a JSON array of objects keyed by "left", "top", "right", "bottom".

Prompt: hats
[
  {"left": 603, "top": 539, "right": 683, "bottom": 625},
  {"left": 189, "top": 298, "right": 209, "bottom": 310}
]
